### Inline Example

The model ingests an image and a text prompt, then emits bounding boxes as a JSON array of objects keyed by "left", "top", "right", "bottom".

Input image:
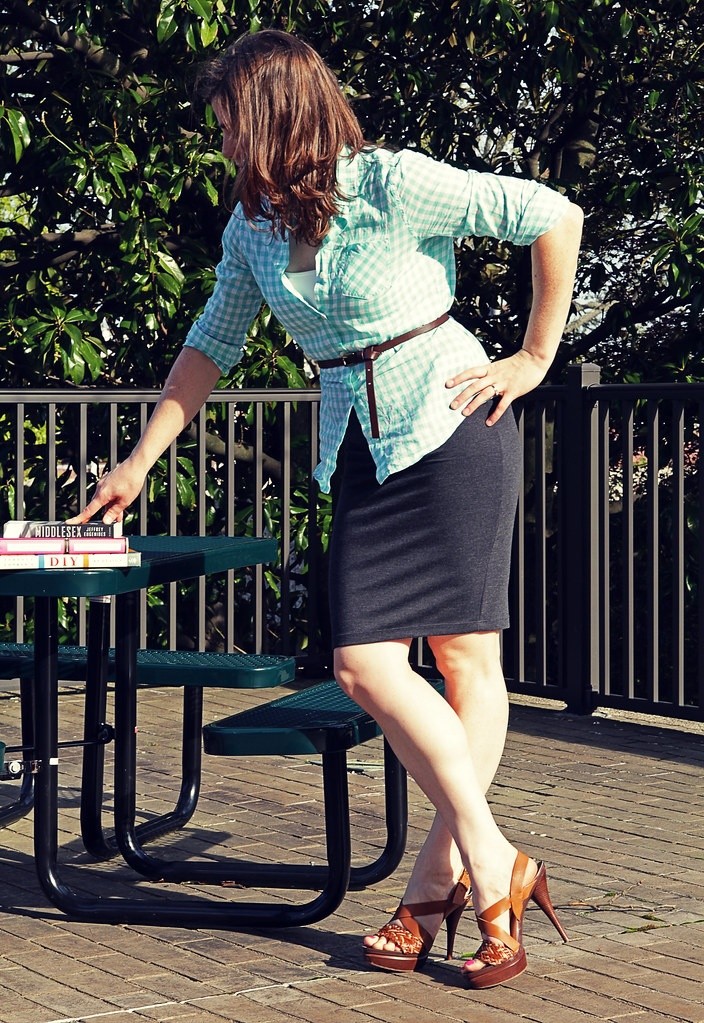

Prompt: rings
[{"left": 489, "top": 385, "right": 499, "bottom": 398}]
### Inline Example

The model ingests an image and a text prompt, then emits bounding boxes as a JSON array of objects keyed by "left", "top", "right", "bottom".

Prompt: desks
[{"left": 0, "top": 534, "right": 280, "bottom": 930}]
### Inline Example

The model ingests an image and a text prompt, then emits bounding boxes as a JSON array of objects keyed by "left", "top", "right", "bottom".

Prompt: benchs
[{"left": 0, "top": 642, "right": 443, "bottom": 929}]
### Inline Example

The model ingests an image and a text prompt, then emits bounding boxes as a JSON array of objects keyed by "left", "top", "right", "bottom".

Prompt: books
[{"left": 0, "top": 520, "right": 141, "bottom": 570}]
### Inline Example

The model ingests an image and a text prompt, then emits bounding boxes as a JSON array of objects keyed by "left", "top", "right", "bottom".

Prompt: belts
[{"left": 318, "top": 313, "right": 449, "bottom": 438}]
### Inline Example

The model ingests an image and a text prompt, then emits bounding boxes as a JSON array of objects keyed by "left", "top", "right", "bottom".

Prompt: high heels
[
  {"left": 461, "top": 851, "right": 569, "bottom": 990},
  {"left": 362, "top": 868, "right": 473, "bottom": 973}
]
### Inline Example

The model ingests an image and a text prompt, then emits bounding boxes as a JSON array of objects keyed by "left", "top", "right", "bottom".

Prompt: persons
[{"left": 64, "top": 29, "right": 584, "bottom": 989}]
